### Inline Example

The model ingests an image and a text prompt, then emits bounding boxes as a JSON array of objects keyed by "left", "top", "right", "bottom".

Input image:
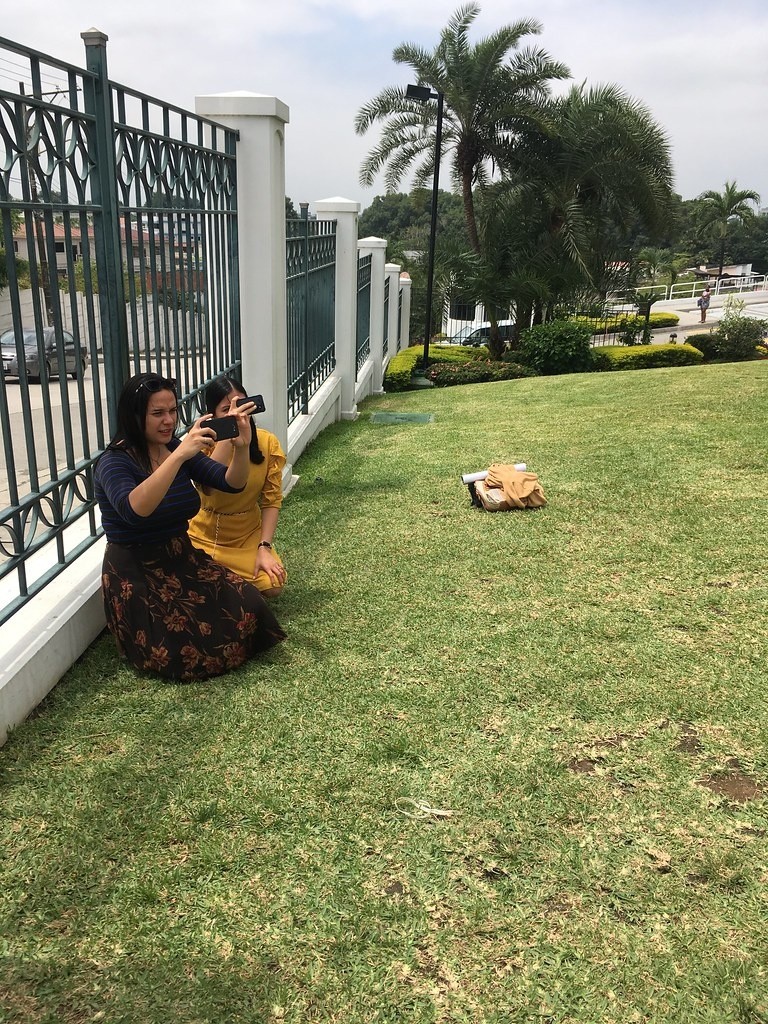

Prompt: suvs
[{"left": 434, "top": 321, "right": 519, "bottom": 352}]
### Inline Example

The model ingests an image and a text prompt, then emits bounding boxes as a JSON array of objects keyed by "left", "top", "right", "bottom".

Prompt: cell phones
[
  {"left": 236, "top": 395, "right": 265, "bottom": 415},
  {"left": 200, "top": 416, "right": 239, "bottom": 444}
]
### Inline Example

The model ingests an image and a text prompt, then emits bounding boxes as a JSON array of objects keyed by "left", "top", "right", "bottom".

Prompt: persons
[
  {"left": 91, "top": 372, "right": 288, "bottom": 682},
  {"left": 187, "top": 375, "right": 287, "bottom": 598},
  {"left": 698, "top": 285, "right": 711, "bottom": 323}
]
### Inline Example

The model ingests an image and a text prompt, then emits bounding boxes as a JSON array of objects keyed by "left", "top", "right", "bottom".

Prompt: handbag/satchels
[{"left": 468, "top": 479, "right": 513, "bottom": 512}]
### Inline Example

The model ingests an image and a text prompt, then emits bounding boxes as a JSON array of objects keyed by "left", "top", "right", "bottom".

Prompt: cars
[{"left": 0, "top": 326, "right": 88, "bottom": 382}]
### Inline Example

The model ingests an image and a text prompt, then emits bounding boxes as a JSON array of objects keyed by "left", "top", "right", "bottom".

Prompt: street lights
[{"left": 402, "top": 84, "right": 444, "bottom": 368}]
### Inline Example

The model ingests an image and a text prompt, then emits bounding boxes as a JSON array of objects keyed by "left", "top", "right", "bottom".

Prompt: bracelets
[{"left": 258, "top": 541, "right": 272, "bottom": 549}]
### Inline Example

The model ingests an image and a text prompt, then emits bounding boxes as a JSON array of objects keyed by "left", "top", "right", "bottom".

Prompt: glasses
[{"left": 135, "top": 378, "right": 178, "bottom": 393}]
